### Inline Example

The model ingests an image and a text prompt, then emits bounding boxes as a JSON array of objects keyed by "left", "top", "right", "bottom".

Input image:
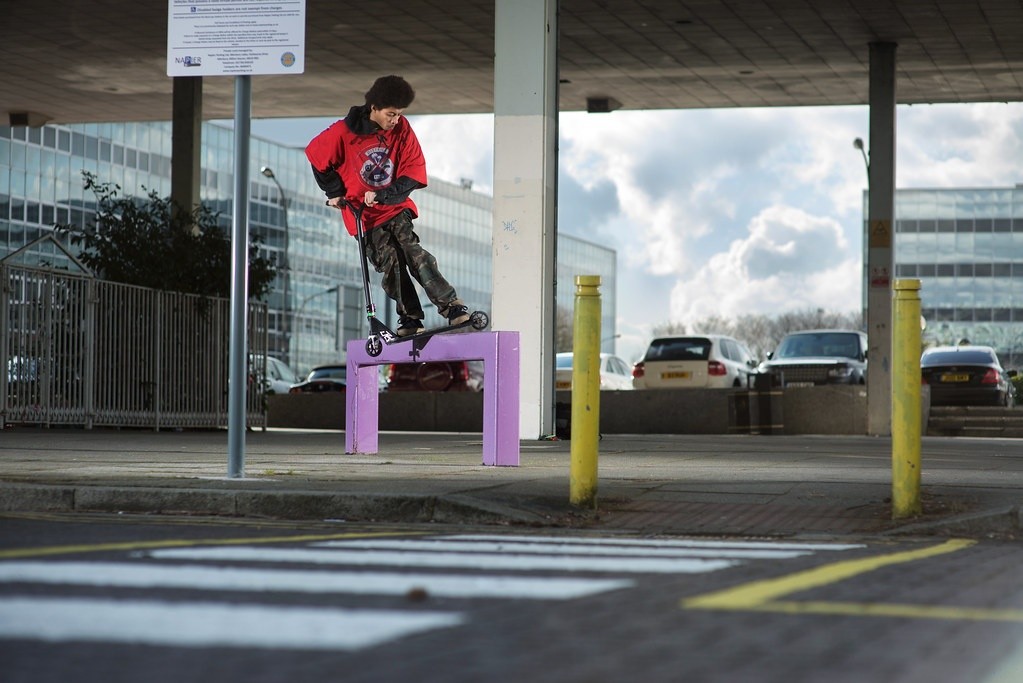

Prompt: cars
[
  {"left": 757, "top": 330, "right": 869, "bottom": 388},
  {"left": 632, "top": 334, "right": 758, "bottom": 388},
  {"left": 556, "top": 352, "right": 633, "bottom": 390},
  {"left": 7, "top": 356, "right": 76, "bottom": 400},
  {"left": 288, "top": 363, "right": 346, "bottom": 392},
  {"left": 921, "top": 345, "right": 1017, "bottom": 407},
  {"left": 249, "top": 353, "right": 301, "bottom": 395}
]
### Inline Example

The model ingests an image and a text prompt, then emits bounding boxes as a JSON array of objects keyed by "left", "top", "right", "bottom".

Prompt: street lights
[
  {"left": 261, "top": 166, "right": 289, "bottom": 362},
  {"left": 855, "top": 138, "right": 870, "bottom": 180}
]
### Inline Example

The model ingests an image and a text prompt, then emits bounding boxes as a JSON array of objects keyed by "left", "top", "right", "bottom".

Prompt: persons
[{"left": 305, "top": 75, "right": 470, "bottom": 337}]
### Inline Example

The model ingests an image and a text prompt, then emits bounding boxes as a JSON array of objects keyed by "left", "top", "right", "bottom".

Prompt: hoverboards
[{"left": 325, "top": 197, "right": 488, "bottom": 357}]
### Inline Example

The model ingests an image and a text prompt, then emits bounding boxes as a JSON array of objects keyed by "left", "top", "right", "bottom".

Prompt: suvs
[{"left": 387, "top": 361, "right": 469, "bottom": 390}]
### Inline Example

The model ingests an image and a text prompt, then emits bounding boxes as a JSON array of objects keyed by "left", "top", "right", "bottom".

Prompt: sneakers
[
  {"left": 396, "top": 315, "right": 425, "bottom": 336},
  {"left": 448, "top": 304, "right": 468, "bottom": 325}
]
